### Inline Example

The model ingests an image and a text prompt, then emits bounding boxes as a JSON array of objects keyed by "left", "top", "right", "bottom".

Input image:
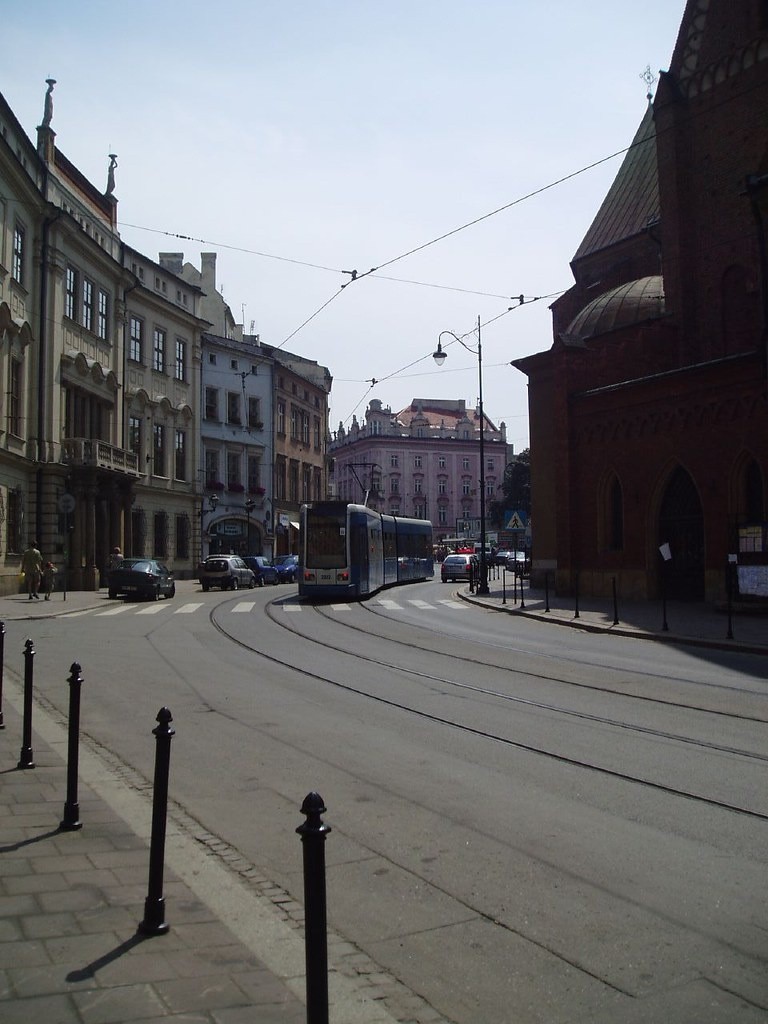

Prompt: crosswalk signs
[{"left": 504, "top": 510, "right": 526, "bottom": 532}]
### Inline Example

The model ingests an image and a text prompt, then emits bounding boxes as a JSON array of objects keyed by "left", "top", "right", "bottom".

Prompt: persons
[
  {"left": 110, "top": 546, "right": 125, "bottom": 573},
  {"left": 39, "top": 562, "right": 60, "bottom": 602},
  {"left": 20, "top": 539, "right": 41, "bottom": 599}
]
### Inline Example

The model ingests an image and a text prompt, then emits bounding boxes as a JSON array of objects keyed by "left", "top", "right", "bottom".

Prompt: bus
[
  {"left": 442, "top": 537, "right": 478, "bottom": 554},
  {"left": 296, "top": 502, "right": 434, "bottom": 599}
]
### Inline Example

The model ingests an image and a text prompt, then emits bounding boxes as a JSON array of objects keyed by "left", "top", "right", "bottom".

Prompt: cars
[
  {"left": 269, "top": 554, "right": 301, "bottom": 583},
  {"left": 241, "top": 554, "right": 280, "bottom": 585},
  {"left": 106, "top": 558, "right": 175, "bottom": 601},
  {"left": 475, "top": 542, "right": 528, "bottom": 572},
  {"left": 441, "top": 555, "right": 477, "bottom": 583},
  {"left": 201, "top": 558, "right": 256, "bottom": 592},
  {"left": 203, "top": 555, "right": 238, "bottom": 561}
]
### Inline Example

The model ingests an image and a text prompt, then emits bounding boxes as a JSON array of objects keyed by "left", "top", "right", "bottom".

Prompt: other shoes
[
  {"left": 45, "top": 596, "right": 50, "bottom": 600},
  {"left": 29, "top": 592, "right": 39, "bottom": 600}
]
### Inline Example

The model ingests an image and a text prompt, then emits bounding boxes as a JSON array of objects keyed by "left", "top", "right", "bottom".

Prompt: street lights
[{"left": 430, "top": 315, "right": 492, "bottom": 595}]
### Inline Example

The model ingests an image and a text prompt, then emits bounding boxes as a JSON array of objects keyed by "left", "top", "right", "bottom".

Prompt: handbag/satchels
[{"left": 18, "top": 570, "right": 26, "bottom": 582}]
